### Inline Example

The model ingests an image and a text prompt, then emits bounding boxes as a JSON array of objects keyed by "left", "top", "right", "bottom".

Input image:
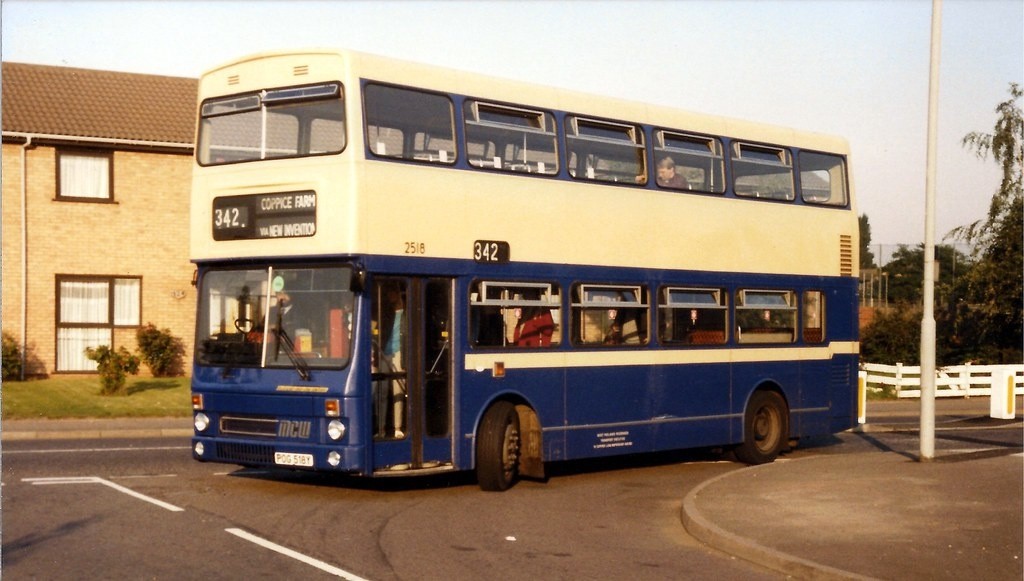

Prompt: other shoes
[
  {"left": 394, "top": 430, "right": 405, "bottom": 440},
  {"left": 373, "top": 432, "right": 384, "bottom": 439}
]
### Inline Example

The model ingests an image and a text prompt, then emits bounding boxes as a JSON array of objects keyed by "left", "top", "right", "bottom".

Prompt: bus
[{"left": 191, "top": 49, "right": 863, "bottom": 491}]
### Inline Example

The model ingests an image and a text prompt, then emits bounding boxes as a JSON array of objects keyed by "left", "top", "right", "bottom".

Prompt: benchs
[{"left": 684, "top": 326, "right": 826, "bottom": 346}]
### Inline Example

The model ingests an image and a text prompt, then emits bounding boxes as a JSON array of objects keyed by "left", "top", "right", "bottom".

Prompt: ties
[{"left": 281, "top": 308, "right": 285, "bottom": 316}]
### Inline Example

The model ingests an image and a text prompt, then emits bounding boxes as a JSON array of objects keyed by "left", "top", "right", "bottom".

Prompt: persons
[
  {"left": 656, "top": 156, "right": 688, "bottom": 189},
  {"left": 513, "top": 289, "right": 554, "bottom": 347},
  {"left": 374, "top": 286, "right": 408, "bottom": 440},
  {"left": 261, "top": 282, "right": 306, "bottom": 340}
]
